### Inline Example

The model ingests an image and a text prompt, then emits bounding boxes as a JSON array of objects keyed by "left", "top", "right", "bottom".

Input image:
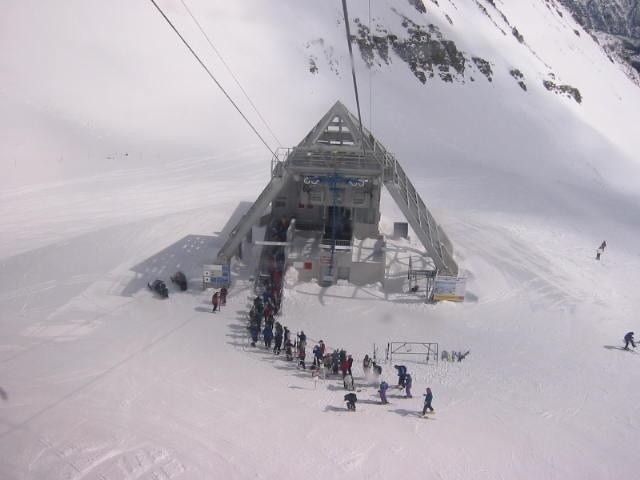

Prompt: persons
[
  {"left": 624, "top": 331, "right": 636, "bottom": 349},
  {"left": 220, "top": 286, "right": 227, "bottom": 304},
  {"left": 422, "top": 387, "right": 433, "bottom": 416},
  {"left": 211, "top": 292, "right": 219, "bottom": 311},
  {"left": 246, "top": 213, "right": 412, "bottom": 413}
]
